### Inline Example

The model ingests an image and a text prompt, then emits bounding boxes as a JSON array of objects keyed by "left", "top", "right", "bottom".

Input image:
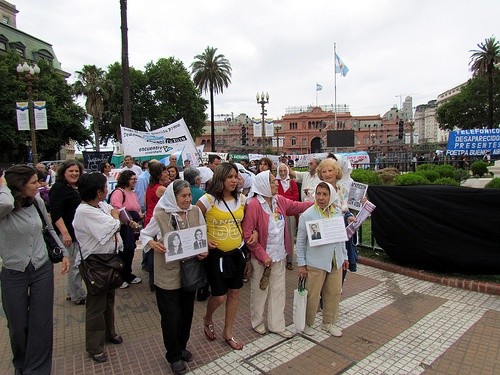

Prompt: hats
[
  {"left": 150, "top": 159, "right": 160, "bottom": 164},
  {"left": 149, "top": 163, "right": 166, "bottom": 176}
]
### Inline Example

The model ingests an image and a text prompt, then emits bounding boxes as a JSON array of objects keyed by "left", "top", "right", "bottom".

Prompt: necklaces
[{"left": 87, "top": 203, "right": 101, "bottom": 208}]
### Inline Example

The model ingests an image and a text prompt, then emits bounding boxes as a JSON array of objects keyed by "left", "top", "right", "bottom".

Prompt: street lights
[
  {"left": 256, "top": 91, "right": 270, "bottom": 152},
  {"left": 18, "top": 61, "right": 40, "bottom": 165}
]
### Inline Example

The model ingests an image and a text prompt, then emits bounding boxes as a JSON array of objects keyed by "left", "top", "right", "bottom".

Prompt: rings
[{"left": 267, "top": 264, "right": 269, "bottom": 267}]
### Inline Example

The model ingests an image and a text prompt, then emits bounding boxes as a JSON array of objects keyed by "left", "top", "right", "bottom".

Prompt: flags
[
  {"left": 335, "top": 53, "right": 349, "bottom": 76},
  {"left": 316, "top": 84, "right": 322, "bottom": 91}
]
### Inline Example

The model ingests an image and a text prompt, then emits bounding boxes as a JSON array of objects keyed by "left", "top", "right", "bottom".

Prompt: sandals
[
  {"left": 224, "top": 336, "right": 243, "bottom": 350},
  {"left": 203, "top": 318, "right": 216, "bottom": 341}
]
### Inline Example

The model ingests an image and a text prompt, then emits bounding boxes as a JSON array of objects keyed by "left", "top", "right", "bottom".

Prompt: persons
[
  {"left": 167, "top": 233, "right": 183, "bottom": 256},
  {"left": 374, "top": 152, "right": 493, "bottom": 172},
  {"left": 310, "top": 224, "right": 322, "bottom": 240},
  {"left": 31, "top": 154, "right": 366, "bottom": 375},
  {"left": 193, "top": 229, "right": 207, "bottom": 250},
  {"left": 0, "top": 165, "right": 70, "bottom": 375}
]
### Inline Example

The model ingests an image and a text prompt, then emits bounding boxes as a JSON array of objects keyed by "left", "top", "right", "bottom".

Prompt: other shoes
[
  {"left": 65, "top": 296, "right": 71, "bottom": 301},
  {"left": 303, "top": 324, "right": 314, "bottom": 336},
  {"left": 74, "top": 298, "right": 87, "bottom": 305},
  {"left": 130, "top": 278, "right": 142, "bottom": 284},
  {"left": 254, "top": 322, "right": 266, "bottom": 335},
  {"left": 91, "top": 351, "right": 107, "bottom": 362},
  {"left": 320, "top": 323, "right": 343, "bottom": 336},
  {"left": 171, "top": 359, "right": 187, "bottom": 375},
  {"left": 286, "top": 262, "right": 294, "bottom": 269},
  {"left": 179, "top": 348, "right": 192, "bottom": 362},
  {"left": 142, "top": 266, "right": 149, "bottom": 272},
  {"left": 106, "top": 333, "right": 123, "bottom": 344},
  {"left": 276, "top": 329, "right": 293, "bottom": 338},
  {"left": 119, "top": 282, "right": 129, "bottom": 288},
  {"left": 243, "top": 274, "right": 249, "bottom": 283},
  {"left": 199, "top": 291, "right": 210, "bottom": 301}
]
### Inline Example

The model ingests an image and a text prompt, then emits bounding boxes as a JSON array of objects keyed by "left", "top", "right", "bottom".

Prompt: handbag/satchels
[
  {"left": 293, "top": 276, "right": 308, "bottom": 331},
  {"left": 180, "top": 257, "right": 205, "bottom": 288},
  {"left": 33, "top": 196, "right": 64, "bottom": 263},
  {"left": 85, "top": 255, "right": 122, "bottom": 291}
]
